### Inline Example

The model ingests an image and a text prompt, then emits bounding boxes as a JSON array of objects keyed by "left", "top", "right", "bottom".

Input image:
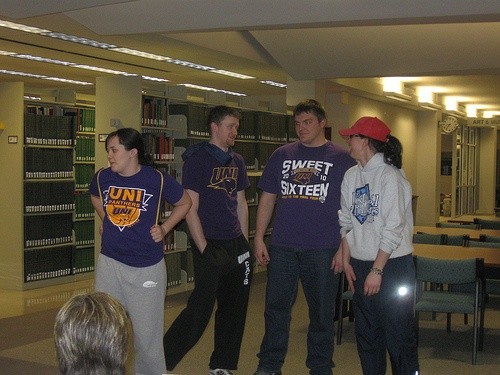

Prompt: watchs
[{"left": 370, "top": 268, "right": 384, "bottom": 275}]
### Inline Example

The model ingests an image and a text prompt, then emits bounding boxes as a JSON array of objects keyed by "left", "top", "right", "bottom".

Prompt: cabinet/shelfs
[{"left": 0, "top": 82, "right": 333, "bottom": 292}]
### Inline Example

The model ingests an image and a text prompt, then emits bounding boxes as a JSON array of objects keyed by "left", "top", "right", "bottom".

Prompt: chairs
[{"left": 335, "top": 218, "right": 500, "bottom": 365}]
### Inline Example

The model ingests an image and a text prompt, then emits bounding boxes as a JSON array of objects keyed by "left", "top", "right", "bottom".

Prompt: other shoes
[
  {"left": 252, "top": 368, "right": 282, "bottom": 375},
  {"left": 208, "top": 368, "right": 234, "bottom": 375},
  {"left": 309, "top": 369, "right": 334, "bottom": 375}
]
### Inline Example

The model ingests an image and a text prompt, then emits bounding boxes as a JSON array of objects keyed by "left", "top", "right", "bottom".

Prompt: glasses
[{"left": 349, "top": 135, "right": 365, "bottom": 140}]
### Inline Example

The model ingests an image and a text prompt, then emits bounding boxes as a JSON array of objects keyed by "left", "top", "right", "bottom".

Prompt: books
[{"left": 23, "top": 98, "right": 300, "bottom": 289}]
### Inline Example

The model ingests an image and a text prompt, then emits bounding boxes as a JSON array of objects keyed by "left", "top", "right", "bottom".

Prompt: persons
[
  {"left": 88, "top": 128, "right": 191, "bottom": 375},
  {"left": 163, "top": 105, "right": 253, "bottom": 375},
  {"left": 338, "top": 116, "right": 421, "bottom": 375},
  {"left": 253, "top": 99, "right": 358, "bottom": 375},
  {"left": 53, "top": 291, "right": 132, "bottom": 375}
]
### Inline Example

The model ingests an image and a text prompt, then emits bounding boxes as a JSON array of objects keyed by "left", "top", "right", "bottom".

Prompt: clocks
[{"left": 438, "top": 116, "right": 458, "bottom": 133}]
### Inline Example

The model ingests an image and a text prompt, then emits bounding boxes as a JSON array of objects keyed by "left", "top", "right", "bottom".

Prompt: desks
[
  {"left": 448, "top": 214, "right": 500, "bottom": 226},
  {"left": 413, "top": 226, "right": 500, "bottom": 242},
  {"left": 412, "top": 244, "right": 500, "bottom": 267}
]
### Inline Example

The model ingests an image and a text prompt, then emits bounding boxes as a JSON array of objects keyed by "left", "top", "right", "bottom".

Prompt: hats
[{"left": 338, "top": 116, "right": 392, "bottom": 143}]
